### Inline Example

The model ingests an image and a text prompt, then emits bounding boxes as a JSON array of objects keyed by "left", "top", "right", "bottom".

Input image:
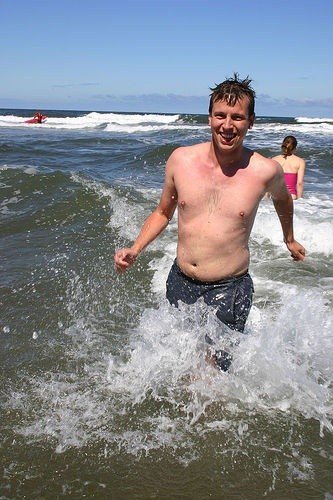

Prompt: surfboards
[{"left": 23, "top": 115, "right": 46, "bottom": 123}]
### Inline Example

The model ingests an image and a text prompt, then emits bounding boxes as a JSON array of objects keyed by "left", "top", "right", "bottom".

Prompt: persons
[
  {"left": 34, "top": 112, "right": 40, "bottom": 118},
  {"left": 114, "top": 73, "right": 307, "bottom": 372},
  {"left": 266, "top": 136, "right": 305, "bottom": 200}
]
